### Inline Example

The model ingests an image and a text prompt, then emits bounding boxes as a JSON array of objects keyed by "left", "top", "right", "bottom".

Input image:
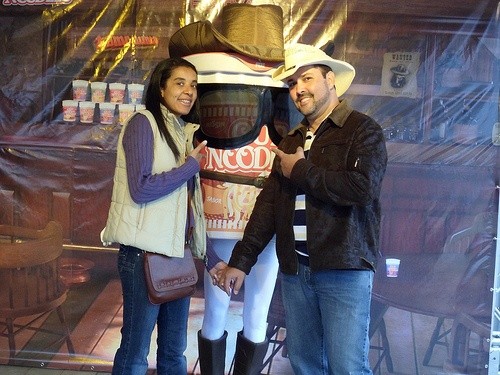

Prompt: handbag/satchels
[{"left": 144, "top": 244, "right": 198, "bottom": 305}]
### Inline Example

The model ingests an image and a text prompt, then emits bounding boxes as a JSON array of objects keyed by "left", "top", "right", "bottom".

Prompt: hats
[
  {"left": 272, "top": 41, "right": 356, "bottom": 97},
  {"left": 168, "top": 3, "right": 335, "bottom": 63}
]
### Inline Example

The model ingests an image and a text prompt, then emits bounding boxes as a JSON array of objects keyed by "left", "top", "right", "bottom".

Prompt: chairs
[
  {"left": 267, "top": 268, "right": 397, "bottom": 375},
  {"left": 0, "top": 221, "right": 75, "bottom": 361},
  {"left": 418, "top": 210, "right": 497, "bottom": 375}
]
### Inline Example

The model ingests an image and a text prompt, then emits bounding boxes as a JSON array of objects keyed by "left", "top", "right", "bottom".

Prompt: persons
[
  {"left": 101, "top": 56, "right": 234, "bottom": 375},
  {"left": 218, "top": 45, "right": 387, "bottom": 375},
  {"left": 168, "top": 3, "right": 355, "bottom": 375}
]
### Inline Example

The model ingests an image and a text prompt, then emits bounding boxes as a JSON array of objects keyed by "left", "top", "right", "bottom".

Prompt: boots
[
  {"left": 233, "top": 330, "right": 268, "bottom": 375},
  {"left": 197, "top": 329, "right": 228, "bottom": 375}
]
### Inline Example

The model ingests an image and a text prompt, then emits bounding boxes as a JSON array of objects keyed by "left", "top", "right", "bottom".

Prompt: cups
[
  {"left": 62, "top": 80, "right": 145, "bottom": 126},
  {"left": 385, "top": 259, "right": 401, "bottom": 278}
]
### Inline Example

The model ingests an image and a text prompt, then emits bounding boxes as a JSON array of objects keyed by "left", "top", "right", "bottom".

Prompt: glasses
[{"left": 181, "top": 82, "right": 307, "bottom": 150}]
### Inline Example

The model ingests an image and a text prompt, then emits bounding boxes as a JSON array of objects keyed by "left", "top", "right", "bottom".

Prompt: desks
[{"left": 371, "top": 251, "right": 494, "bottom": 365}]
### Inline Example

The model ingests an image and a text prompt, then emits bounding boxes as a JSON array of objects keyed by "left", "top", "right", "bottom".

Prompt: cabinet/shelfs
[{"left": 0, "top": 5, "right": 500, "bottom": 272}]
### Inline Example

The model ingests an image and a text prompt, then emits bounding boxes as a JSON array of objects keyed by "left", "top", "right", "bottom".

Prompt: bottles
[
  {"left": 454, "top": 110, "right": 478, "bottom": 145},
  {"left": 439, "top": 109, "right": 451, "bottom": 142}
]
